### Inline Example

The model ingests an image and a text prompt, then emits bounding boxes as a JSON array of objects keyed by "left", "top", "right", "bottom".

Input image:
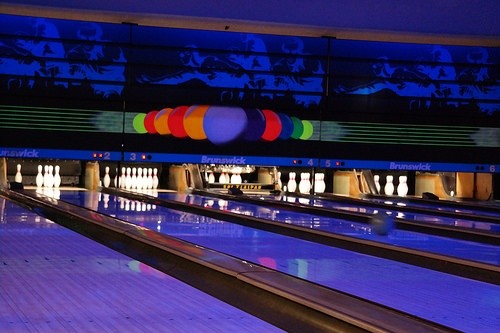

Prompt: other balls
[{"left": 371, "top": 213, "right": 397, "bottom": 236}]
